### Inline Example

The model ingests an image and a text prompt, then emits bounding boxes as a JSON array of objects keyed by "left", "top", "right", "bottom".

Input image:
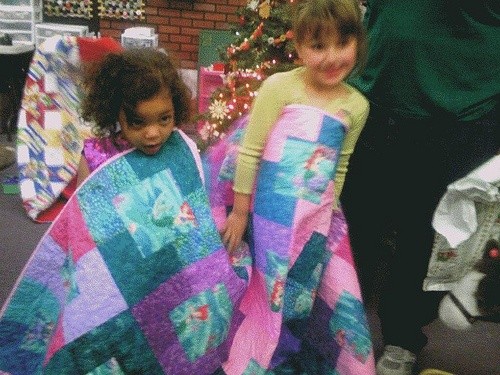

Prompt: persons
[
  {"left": 341, "top": 0, "right": 498, "bottom": 375},
  {"left": 76, "top": 46, "right": 194, "bottom": 193},
  {"left": 220, "top": 0, "right": 372, "bottom": 255}
]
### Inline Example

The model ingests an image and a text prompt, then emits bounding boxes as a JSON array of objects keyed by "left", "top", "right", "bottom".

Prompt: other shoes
[{"left": 376, "top": 344, "right": 415, "bottom": 375}]
